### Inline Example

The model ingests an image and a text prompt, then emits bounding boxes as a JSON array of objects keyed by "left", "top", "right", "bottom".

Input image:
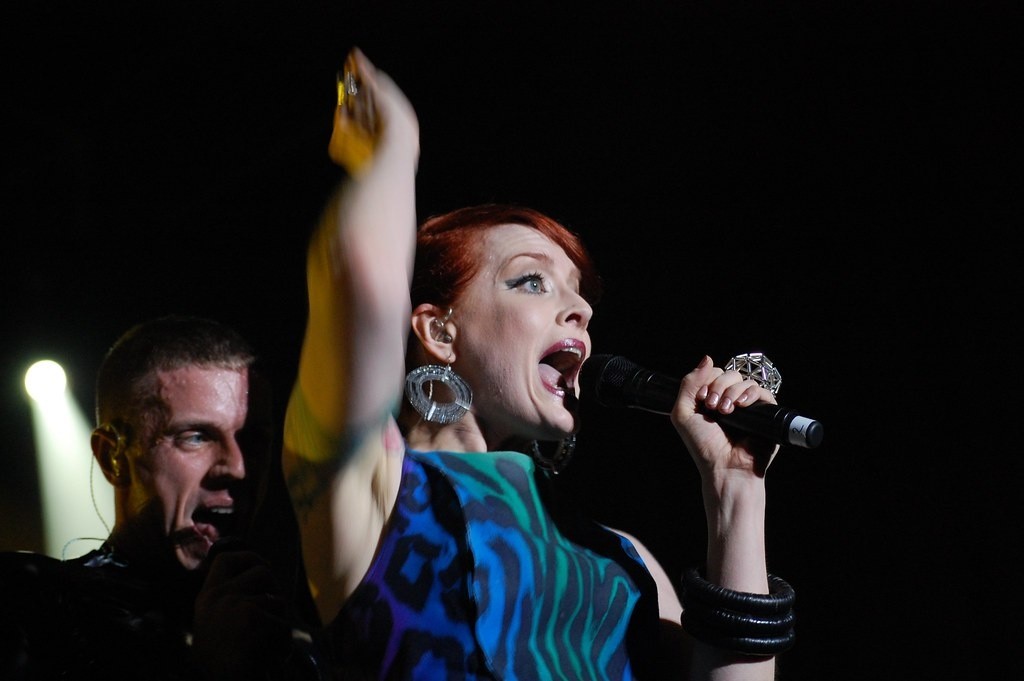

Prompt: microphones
[
  {"left": 578, "top": 355, "right": 824, "bottom": 449},
  {"left": 205, "top": 537, "right": 318, "bottom": 668}
]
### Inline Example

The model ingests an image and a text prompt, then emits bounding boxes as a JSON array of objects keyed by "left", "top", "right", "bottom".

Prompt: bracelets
[{"left": 681, "top": 568, "right": 795, "bottom": 655}]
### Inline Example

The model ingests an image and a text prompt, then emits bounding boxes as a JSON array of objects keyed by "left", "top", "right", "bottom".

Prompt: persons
[
  {"left": 0, "top": 317, "right": 277, "bottom": 681},
  {"left": 281, "top": 49, "right": 780, "bottom": 681}
]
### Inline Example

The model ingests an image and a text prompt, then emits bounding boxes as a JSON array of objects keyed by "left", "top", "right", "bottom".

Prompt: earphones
[
  {"left": 107, "top": 448, "right": 123, "bottom": 477},
  {"left": 429, "top": 319, "right": 453, "bottom": 343}
]
